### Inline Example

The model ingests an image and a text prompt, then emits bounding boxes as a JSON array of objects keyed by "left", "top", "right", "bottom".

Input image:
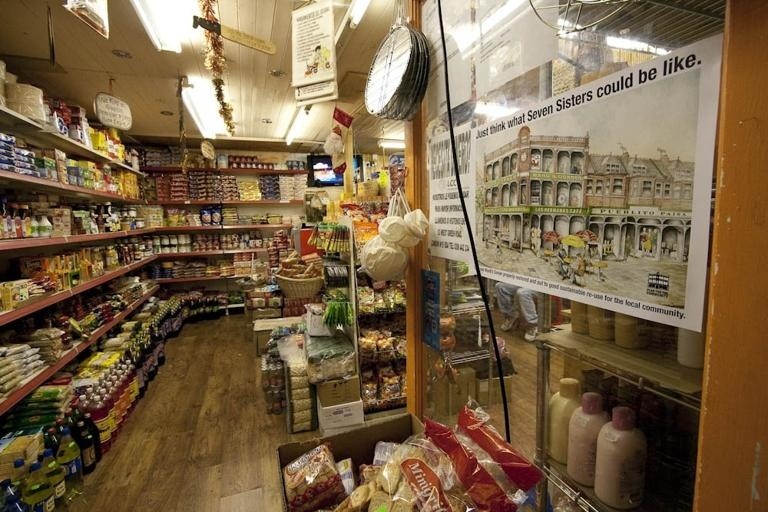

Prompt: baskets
[{"left": 275, "top": 274, "right": 324, "bottom": 300}]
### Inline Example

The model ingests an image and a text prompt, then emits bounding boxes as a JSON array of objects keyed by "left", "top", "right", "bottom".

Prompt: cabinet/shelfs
[
  {"left": 332, "top": 218, "right": 408, "bottom": 409},
  {"left": 0, "top": 102, "right": 160, "bottom": 478},
  {"left": 525, "top": 1, "right": 702, "bottom": 512},
  {"left": 140, "top": 160, "right": 311, "bottom": 316}
]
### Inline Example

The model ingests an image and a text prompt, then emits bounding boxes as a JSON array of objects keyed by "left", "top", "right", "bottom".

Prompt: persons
[
  {"left": 495, "top": 282, "right": 539, "bottom": 342},
  {"left": 556, "top": 249, "right": 585, "bottom": 284},
  {"left": 640, "top": 228, "right": 657, "bottom": 258}
]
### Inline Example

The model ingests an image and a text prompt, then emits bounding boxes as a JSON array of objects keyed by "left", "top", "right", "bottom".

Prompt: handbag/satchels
[{"left": 360, "top": 186, "right": 430, "bottom": 280}]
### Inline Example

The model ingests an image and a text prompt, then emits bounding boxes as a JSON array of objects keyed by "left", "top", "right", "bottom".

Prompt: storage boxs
[
  {"left": 313, "top": 375, "right": 365, "bottom": 427},
  {"left": 437, "top": 373, "right": 513, "bottom": 414},
  {"left": 275, "top": 411, "right": 430, "bottom": 512}
]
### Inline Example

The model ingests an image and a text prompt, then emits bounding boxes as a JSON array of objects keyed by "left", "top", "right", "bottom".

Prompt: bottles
[
  {"left": 0, "top": 323, "right": 169, "bottom": 507},
  {"left": 0, "top": 197, "right": 54, "bottom": 238},
  {"left": 183, "top": 296, "right": 224, "bottom": 324},
  {"left": 90, "top": 239, "right": 135, "bottom": 277},
  {"left": 154, "top": 300, "right": 184, "bottom": 334},
  {"left": 547, "top": 377, "right": 648, "bottom": 509}
]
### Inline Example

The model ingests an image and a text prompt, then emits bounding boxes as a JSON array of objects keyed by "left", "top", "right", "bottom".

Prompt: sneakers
[
  {"left": 523, "top": 320, "right": 539, "bottom": 342},
  {"left": 500, "top": 309, "right": 520, "bottom": 332}
]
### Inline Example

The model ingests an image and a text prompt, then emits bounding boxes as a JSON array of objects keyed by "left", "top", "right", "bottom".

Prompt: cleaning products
[
  {"left": 594, "top": 408, "right": 648, "bottom": 510},
  {"left": 566, "top": 394, "right": 611, "bottom": 488},
  {"left": 549, "top": 377, "right": 582, "bottom": 466}
]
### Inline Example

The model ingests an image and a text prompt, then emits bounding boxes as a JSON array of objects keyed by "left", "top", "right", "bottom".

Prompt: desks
[{"left": 253, "top": 316, "right": 304, "bottom": 353}]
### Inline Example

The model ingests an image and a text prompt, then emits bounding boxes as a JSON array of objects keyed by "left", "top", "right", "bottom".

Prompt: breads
[{"left": 350, "top": 444, "right": 466, "bottom": 512}]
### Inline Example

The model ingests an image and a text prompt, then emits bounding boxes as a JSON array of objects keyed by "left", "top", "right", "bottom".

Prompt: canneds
[{"left": 112, "top": 207, "right": 145, "bottom": 229}]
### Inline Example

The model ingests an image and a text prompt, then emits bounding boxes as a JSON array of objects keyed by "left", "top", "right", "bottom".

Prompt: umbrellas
[{"left": 541, "top": 230, "right": 597, "bottom": 250}]
